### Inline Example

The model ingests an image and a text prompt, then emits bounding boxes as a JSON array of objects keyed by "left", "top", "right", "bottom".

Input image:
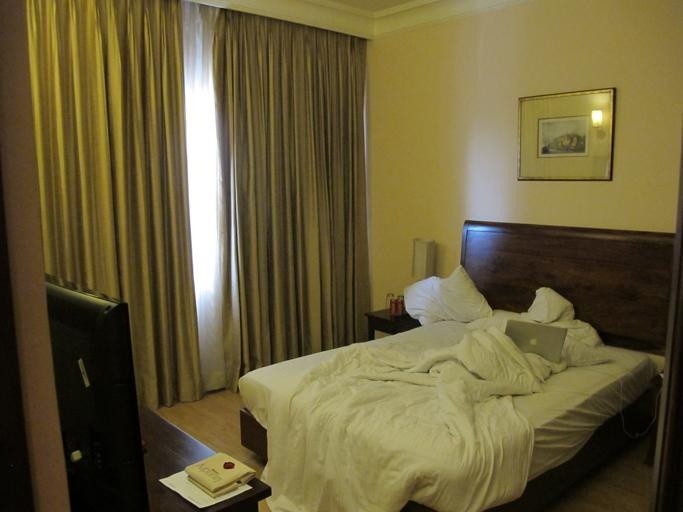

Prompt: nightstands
[{"left": 363, "top": 305, "right": 422, "bottom": 341}]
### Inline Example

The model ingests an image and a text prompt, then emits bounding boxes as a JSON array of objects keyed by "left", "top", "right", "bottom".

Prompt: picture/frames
[{"left": 514, "top": 86, "right": 617, "bottom": 182}]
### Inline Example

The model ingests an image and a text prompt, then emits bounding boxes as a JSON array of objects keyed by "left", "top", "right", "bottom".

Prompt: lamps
[{"left": 410, "top": 237, "right": 435, "bottom": 281}]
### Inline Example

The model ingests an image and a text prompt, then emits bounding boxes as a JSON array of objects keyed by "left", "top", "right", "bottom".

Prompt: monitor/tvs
[{"left": 44, "top": 273, "right": 150, "bottom": 512}]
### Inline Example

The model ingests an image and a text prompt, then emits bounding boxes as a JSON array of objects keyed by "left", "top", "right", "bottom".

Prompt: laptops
[{"left": 505, "top": 319, "right": 568, "bottom": 364}]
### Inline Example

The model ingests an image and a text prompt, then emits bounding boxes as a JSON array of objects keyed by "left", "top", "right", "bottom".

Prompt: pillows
[{"left": 402, "top": 262, "right": 494, "bottom": 328}]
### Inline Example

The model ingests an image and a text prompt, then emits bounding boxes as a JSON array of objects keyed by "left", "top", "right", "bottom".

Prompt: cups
[{"left": 389, "top": 298, "right": 403, "bottom": 316}]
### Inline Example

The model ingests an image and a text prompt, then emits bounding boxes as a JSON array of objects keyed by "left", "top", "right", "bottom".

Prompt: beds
[{"left": 237, "top": 219, "right": 679, "bottom": 512}]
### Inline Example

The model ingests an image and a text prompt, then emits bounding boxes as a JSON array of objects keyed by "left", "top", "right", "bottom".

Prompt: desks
[{"left": 137, "top": 396, "right": 273, "bottom": 512}]
